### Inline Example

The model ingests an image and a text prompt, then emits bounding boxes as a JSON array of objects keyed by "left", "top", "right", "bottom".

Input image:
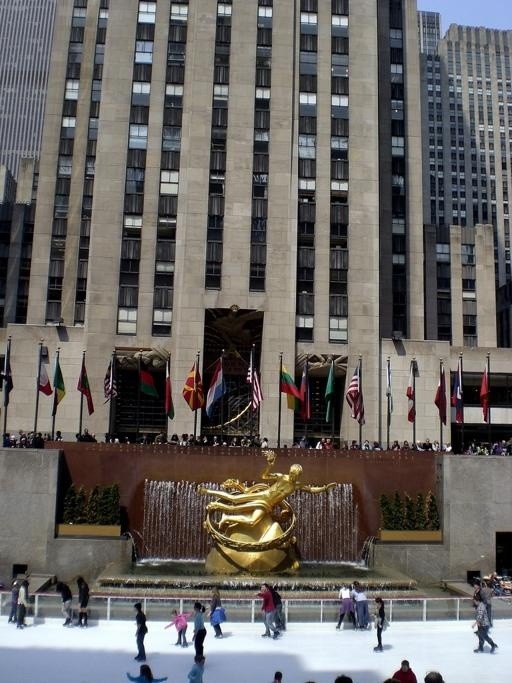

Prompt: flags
[
  {"left": 405, "top": 361, "right": 415, "bottom": 422},
  {"left": 323, "top": 359, "right": 334, "bottom": 421},
  {"left": 51, "top": 352, "right": 66, "bottom": 416},
  {"left": 300, "top": 360, "right": 310, "bottom": 419},
  {"left": 205, "top": 354, "right": 229, "bottom": 418},
  {"left": 78, "top": 356, "right": 95, "bottom": 415},
  {"left": 451, "top": 358, "right": 464, "bottom": 423},
  {"left": 181, "top": 361, "right": 206, "bottom": 411},
  {"left": 385, "top": 360, "right": 393, "bottom": 427},
  {"left": 346, "top": 359, "right": 365, "bottom": 424},
  {"left": 245, "top": 349, "right": 264, "bottom": 412},
  {"left": 479, "top": 359, "right": 489, "bottom": 422},
  {"left": 433, "top": 362, "right": 447, "bottom": 425},
  {"left": 165, "top": 360, "right": 174, "bottom": 419},
  {"left": 279, "top": 360, "right": 303, "bottom": 411},
  {"left": 139, "top": 359, "right": 160, "bottom": 399},
  {"left": 1, "top": 340, "right": 14, "bottom": 408},
  {"left": 102, "top": 360, "right": 118, "bottom": 404},
  {"left": 36, "top": 345, "right": 53, "bottom": 395}
]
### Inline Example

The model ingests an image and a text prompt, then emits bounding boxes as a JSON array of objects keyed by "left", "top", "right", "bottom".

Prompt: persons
[
  {"left": 165, "top": 610, "right": 191, "bottom": 648},
  {"left": 490, "top": 572, "right": 512, "bottom": 597},
  {"left": 256, "top": 584, "right": 285, "bottom": 638},
  {"left": 192, "top": 602, "right": 206, "bottom": 657},
  {"left": 424, "top": 672, "right": 444, "bottom": 683},
  {"left": 7, "top": 579, "right": 20, "bottom": 623},
  {"left": 195, "top": 458, "right": 336, "bottom": 529},
  {"left": 274, "top": 672, "right": 282, "bottom": 683},
  {"left": 207, "top": 587, "right": 227, "bottom": 638},
  {"left": 16, "top": 580, "right": 31, "bottom": 628},
  {"left": 73, "top": 576, "right": 89, "bottom": 627},
  {"left": 300, "top": 436, "right": 334, "bottom": 449},
  {"left": 133, "top": 602, "right": 149, "bottom": 663},
  {"left": 466, "top": 439, "right": 512, "bottom": 456},
  {"left": 392, "top": 660, "right": 417, "bottom": 683},
  {"left": 125, "top": 665, "right": 168, "bottom": 683},
  {"left": 80, "top": 429, "right": 269, "bottom": 449},
  {"left": 342, "top": 439, "right": 452, "bottom": 452},
  {"left": 372, "top": 598, "right": 385, "bottom": 653},
  {"left": 54, "top": 580, "right": 74, "bottom": 625},
  {"left": 337, "top": 581, "right": 370, "bottom": 632},
  {"left": 472, "top": 577, "right": 497, "bottom": 655},
  {"left": 5, "top": 430, "right": 63, "bottom": 449}
]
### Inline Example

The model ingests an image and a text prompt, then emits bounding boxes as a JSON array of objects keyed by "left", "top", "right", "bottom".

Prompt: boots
[
  {"left": 63, "top": 618, "right": 71, "bottom": 625},
  {"left": 76, "top": 612, "right": 87, "bottom": 627}
]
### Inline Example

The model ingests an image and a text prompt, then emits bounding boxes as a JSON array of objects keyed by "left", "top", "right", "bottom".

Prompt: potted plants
[
  {"left": 55, "top": 481, "right": 122, "bottom": 539},
  {"left": 377, "top": 488, "right": 442, "bottom": 544}
]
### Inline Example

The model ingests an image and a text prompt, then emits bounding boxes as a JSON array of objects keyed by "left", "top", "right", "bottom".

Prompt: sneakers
[
  {"left": 134, "top": 655, "right": 146, "bottom": 660},
  {"left": 490, "top": 644, "right": 497, "bottom": 653},
  {"left": 273, "top": 631, "right": 279, "bottom": 638},
  {"left": 9, "top": 619, "right": 26, "bottom": 629},
  {"left": 374, "top": 645, "right": 382, "bottom": 651},
  {"left": 262, "top": 632, "right": 270, "bottom": 636}
]
